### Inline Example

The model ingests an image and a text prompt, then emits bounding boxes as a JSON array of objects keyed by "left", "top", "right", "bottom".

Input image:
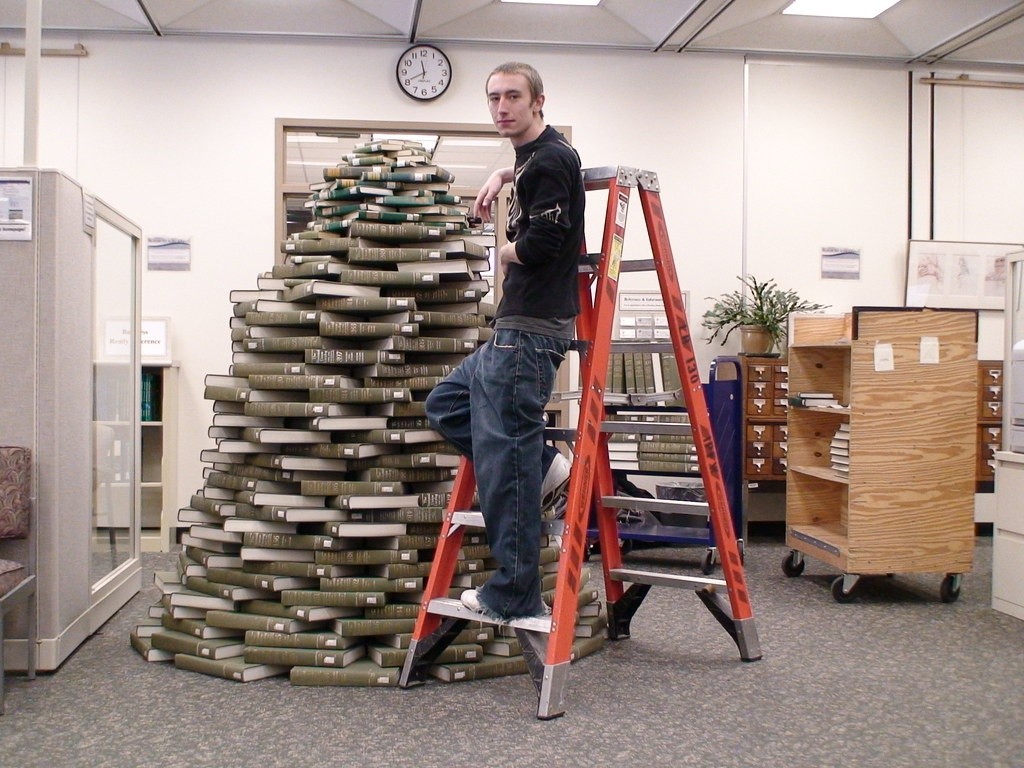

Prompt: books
[
  {"left": 95, "top": 372, "right": 160, "bottom": 421},
  {"left": 788, "top": 390, "right": 839, "bottom": 407},
  {"left": 579, "top": 352, "right": 686, "bottom": 407},
  {"left": 603, "top": 410, "right": 700, "bottom": 472},
  {"left": 830, "top": 423, "right": 850, "bottom": 478},
  {"left": 129, "top": 140, "right": 610, "bottom": 687}
]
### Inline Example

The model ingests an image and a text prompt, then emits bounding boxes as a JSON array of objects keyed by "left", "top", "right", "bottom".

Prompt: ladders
[{"left": 397, "top": 166, "right": 763, "bottom": 722}]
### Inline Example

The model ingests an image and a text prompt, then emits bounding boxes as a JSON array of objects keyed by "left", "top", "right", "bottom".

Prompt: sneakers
[
  {"left": 541, "top": 452, "right": 573, "bottom": 513},
  {"left": 461, "top": 589, "right": 498, "bottom": 616}
]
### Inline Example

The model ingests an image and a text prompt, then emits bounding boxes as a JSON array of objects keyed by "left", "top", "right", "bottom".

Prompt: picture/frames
[{"left": 907, "top": 240, "right": 1023, "bottom": 313}]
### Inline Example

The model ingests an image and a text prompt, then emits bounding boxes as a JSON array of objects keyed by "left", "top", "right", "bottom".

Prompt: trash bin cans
[{"left": 656, "top": 483, "right": 708, "bottom": 548}]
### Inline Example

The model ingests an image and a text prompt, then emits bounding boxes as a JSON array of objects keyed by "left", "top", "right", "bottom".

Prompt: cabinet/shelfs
[
  {"left": 737, "top": 350, "right": 1005, "bottom": 547},
  {"left": 135, "top": 313, "right": 182, "bottom": 554},
  {"left": 782, "top": 307, "right": 978, "bottom": 600}
]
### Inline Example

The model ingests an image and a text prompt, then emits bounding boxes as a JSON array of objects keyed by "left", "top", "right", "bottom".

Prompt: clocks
[{"left": 395, "top": 44, "right": 453, "bottom": 101}]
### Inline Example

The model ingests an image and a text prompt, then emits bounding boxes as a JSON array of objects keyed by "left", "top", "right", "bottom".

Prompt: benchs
[{"left": 0, "top": 445, "right": 38, "bottom": 714}]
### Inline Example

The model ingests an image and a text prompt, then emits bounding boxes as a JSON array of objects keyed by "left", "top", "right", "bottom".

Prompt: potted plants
[{"left": 704, "top": 280, "right": 830, "bottom": 354}]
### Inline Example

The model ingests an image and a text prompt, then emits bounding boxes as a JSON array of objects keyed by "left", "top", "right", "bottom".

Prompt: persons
[{"left": 422, "top": 62, "right": 585, "bottom": 628}]
